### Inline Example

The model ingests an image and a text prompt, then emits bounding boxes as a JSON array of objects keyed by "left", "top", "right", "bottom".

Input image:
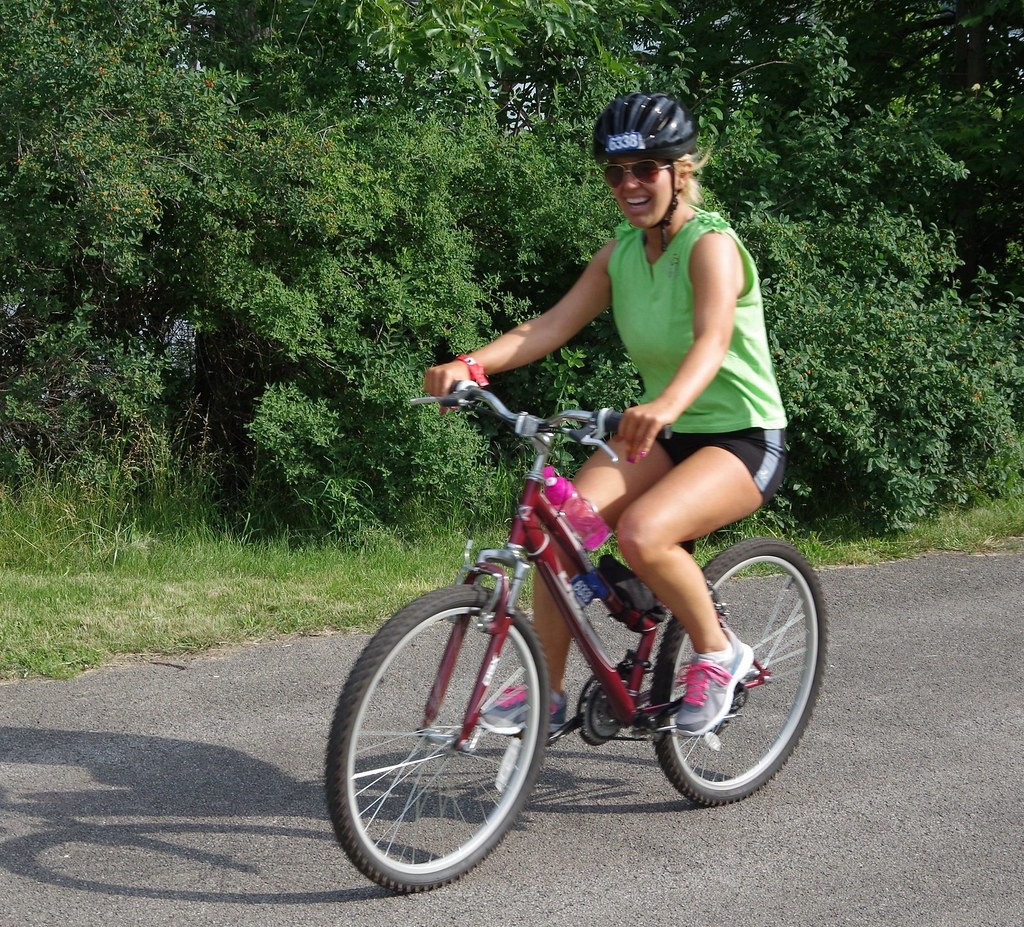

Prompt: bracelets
[{"left": 457, "top": 351, "right": 488, "bottom": 387}]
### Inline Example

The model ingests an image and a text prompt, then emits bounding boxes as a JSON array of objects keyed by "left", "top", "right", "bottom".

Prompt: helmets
[{"left": 593, "top": 94, "right": 698, "bottom": 164}]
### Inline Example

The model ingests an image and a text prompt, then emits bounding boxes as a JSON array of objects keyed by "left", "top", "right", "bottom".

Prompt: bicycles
[{"left": 324, "top": 378, "right": 830, "bottom": 893}]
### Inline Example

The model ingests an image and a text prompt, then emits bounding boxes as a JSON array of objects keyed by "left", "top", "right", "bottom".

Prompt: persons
[{"left": 426, "top": 91, "right": 786, "bottom": 741}]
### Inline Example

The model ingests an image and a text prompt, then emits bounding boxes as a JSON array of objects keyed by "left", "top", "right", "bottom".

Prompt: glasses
[{"left": 604, "top": 159, "right": 672, "bottom": 188}]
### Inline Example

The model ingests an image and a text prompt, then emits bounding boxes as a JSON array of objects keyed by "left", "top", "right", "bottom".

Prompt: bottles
[{"left": 544, "top": 476, "right": 609, "bottom": 551}]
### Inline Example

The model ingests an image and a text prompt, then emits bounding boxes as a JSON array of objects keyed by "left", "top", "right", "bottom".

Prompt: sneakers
[
  {"left": 479, "top": 682, "right": 567, "bottom": 737},
  {"left": 676, "top": 620, "right": 755, "bottom": 736}
]
